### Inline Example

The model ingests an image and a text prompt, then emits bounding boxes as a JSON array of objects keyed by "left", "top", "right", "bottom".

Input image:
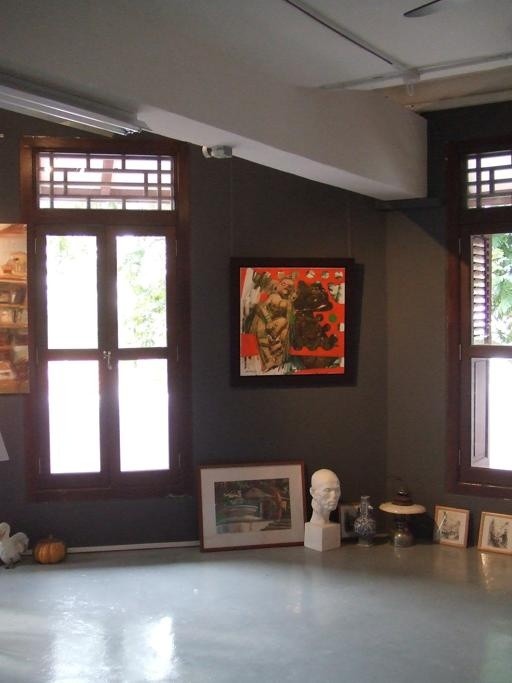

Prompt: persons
[
  {"left": 255, "top": 276, "right": 296, "bottom": 371},
  {"left": 304, "top": 467, "right": 344, "bottom": 553}
]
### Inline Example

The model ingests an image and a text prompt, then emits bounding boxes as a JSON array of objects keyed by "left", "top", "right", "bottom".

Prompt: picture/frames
[
  {"left": 432, "top": 504, "right": 512, "bottom": 555},
  {"left": 229, "top": 255, "right": 365, "bottom": 389},
  {"left": 339, "top": 502, "right": 357, "bottom": 543},
  {"left": 196, "top": 458, "right": 307, "bottom": 554}
]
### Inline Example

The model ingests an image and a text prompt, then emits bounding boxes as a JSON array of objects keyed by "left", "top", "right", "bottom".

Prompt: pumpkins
[{"left": 34, "top": 535, "right": 66, "bottom": 563}]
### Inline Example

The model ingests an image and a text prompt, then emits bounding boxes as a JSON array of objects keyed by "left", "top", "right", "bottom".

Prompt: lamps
[{"left": 0, "top": 73, "right": 151, "bottom": 141}]
